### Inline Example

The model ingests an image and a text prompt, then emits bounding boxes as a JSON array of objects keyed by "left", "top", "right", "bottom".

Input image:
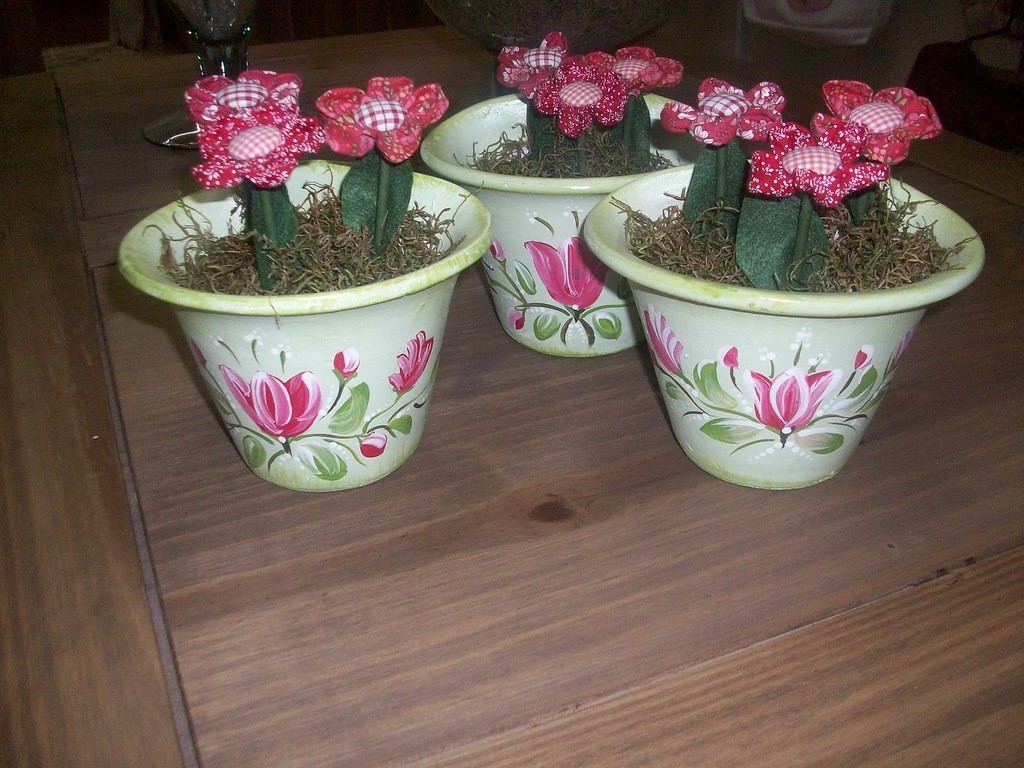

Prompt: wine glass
[{"left": 142, "top": 0, "right": 259, "bottom": 147}]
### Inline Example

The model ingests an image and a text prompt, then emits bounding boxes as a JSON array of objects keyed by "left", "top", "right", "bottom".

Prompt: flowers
[
  {"left": 185, "top": 69, "right": 447, "bottom": 285},
  {"left": 661, "top": 77, "right": 943, "bottom": 294},
  {"left": 639, "top": 311, "right": 912, "bottom": 460},
  {"left": 191, "top": 331, "right": 434, "bottom": 474},
  {"left": 479, "top": 210, "right": 636, "bottom": 349},
  {"left": 497, "top": 31, "right": 684, "bottom": 174}
]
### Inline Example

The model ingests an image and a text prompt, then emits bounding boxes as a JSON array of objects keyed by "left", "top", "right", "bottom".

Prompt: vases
[
  {"left": 118, "top": 160, "right": 494, "bottom": 494},
  {"left": 585, "top": 166, "right": 986, "bottom": 490},
  {"left": 420, "top": 93, "right": 710, "bottom": 356}
]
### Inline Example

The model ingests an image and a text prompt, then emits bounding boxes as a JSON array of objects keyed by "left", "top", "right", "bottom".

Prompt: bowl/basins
[{"left": 427, "top": 0, "right": 672, "bottom": 57}]
